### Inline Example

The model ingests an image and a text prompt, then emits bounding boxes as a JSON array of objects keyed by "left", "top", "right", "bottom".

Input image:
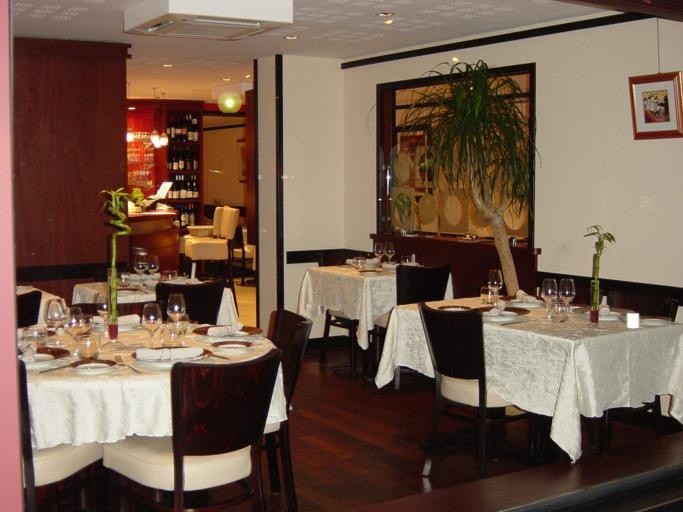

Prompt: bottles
[
  {"left": 167, "top": 145, "right": 197, "bottom": 171},
  {"left": 168, "top": 204, "right": 196, "bottom": 227},
  {"left": 167, "top": 112, "right": 198, "bottom": 142},
  {"left": 166, "top": 175, "right": 199, "bottom": 200}
]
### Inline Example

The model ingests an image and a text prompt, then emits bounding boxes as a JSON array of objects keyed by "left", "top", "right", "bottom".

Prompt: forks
[{"left": 113, "top": 354, "right": 140, "bottom": 376}]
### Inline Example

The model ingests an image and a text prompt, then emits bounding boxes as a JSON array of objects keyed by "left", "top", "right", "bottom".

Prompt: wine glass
[
  {"left": 141, "top": 292, "right": 188, "bottom": 363},
  {"left": 487, "top": 268, "right": 503, "bottom": 306},
  {"left": 133, "top": 253, "right": 148, "bottom": 284},
  {"left": 145, "top": 255, "right": 161, "bottom": 284},
  {"left": 539, "top": 275, "right": 575, "bottom": 325},
  {"left": 94, "top": 289, "right": 111, "bottom": 337},
  {"left": 372, "top": 240, "right": 394, "bottom": 264},
  {"left": 16, "top": 298, "right": 99, "bottom": 374}
]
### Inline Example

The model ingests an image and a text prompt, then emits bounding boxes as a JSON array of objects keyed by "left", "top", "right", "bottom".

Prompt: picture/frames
[
  {"left": 392, "top": 123, "right": 435, "bottom": 198},
  {"left": 626, "top": 71, "right": 683, "bottom": 143}
]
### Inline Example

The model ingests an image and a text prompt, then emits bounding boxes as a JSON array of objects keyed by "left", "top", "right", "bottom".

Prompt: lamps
[
  {"left": 124, "top": 80, "right": 168, "bottom": 150},
  {"left": 216, "top": 90, "right": 242, "bottom": 117}
]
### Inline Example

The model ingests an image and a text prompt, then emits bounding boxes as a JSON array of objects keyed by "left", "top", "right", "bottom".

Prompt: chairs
[
  {"left": 250, "top": 308, "right": 311, "bottom": 508},
  {"left": 314, "top": 248, "right": 373, "bottom": 365},
  {"left": 176, "top": 203, "right": 255, "bottom": 289},
  {"left": 100, "top": 346, "right": 285, "bottom": 511},
  {"left": 359, "top": 260, "right": 454, "bottom": 381},
  {"left": 153, "top": 282, "right": 241, "bottom": 330},
  {"left": 15, "top": 288, "right": 44, "bottom": 327},
  {"left": 416, "top": 298, "right": 537, "bottom": 482},
  {"left": 16, "top": 357, "right": 104, "bottom": 510}
]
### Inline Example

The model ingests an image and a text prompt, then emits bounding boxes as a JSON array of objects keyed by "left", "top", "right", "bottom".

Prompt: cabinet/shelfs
[{"left": 165, "top": 110, "right": 205, "bottom": 277}]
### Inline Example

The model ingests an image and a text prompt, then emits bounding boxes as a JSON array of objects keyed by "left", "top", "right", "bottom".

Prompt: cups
[
  {"left": 626, "top": 312, "right": 640, "bottom": 329},
  {"left": 479, "top": 286, "right": 493, "bottom": 304},
  {"left": 128, "top": 129, "right": 153, "bottom": 188},
  {"left": 117, "top": 271, "right": 131, "bottom": 287},
  {"left": 351, "top": 256, "right": 367, "bottom": 270},
  {"left": 400, "top": 254, "right": 409, "bottom": 264}
]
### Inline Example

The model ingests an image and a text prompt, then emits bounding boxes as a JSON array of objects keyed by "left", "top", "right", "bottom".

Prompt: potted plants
[
  {"left": 582, "top": 223, "right": 616, "bottom": 321},
  {"left": 95, "top": 186, "right": 144, "bottom": 342}
]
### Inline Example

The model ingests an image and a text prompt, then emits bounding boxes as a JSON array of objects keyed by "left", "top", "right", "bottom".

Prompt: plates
[
  {"left": 130, "top": 345, "right": 211, "bottom": 364},
  {"left": 439, "top": 305, "right": 469, "bottom": 312},
  {"left": 358, "top": 270, "right": 378, "bottom": 275},
  {"left": 211, "top": 341, "right": 253, "bottom": 353},
  {"left": 641, "top": 317, "right": 667, "bottom": 328},
  {"left": 192, "top": 325, "right": 263, "bottom": 337},
  {"left": 71, "top": 359, "right": 116, "bottom": 373},
  {"left": 471, "top": 306, "right": 529, "bottom": 317},
  {"left": 499, "top": 294, "right": 517, "bottom": 303}
]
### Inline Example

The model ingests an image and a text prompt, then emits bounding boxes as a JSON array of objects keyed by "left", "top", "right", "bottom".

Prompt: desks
[{"left": 15, "top": 317, "right": 292, "bottom": 457}]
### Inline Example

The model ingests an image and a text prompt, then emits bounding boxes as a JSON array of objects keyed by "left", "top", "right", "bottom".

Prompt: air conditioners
[{"left": 123, "top": 0, "right": 294, "bottom": 43}]
